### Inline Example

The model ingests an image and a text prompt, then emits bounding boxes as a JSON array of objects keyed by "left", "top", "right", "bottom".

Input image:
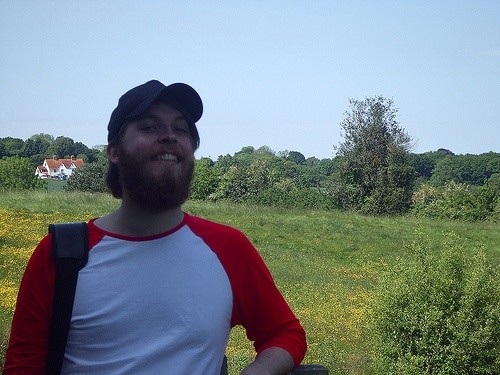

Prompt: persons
[{"left": 2, "top": 80, "right": 307, "bottom": 375}]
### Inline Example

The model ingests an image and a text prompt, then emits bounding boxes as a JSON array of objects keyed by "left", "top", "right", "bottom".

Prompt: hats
[{"left": 107, "top": 80, "right": 204, "bottom": 142}]
